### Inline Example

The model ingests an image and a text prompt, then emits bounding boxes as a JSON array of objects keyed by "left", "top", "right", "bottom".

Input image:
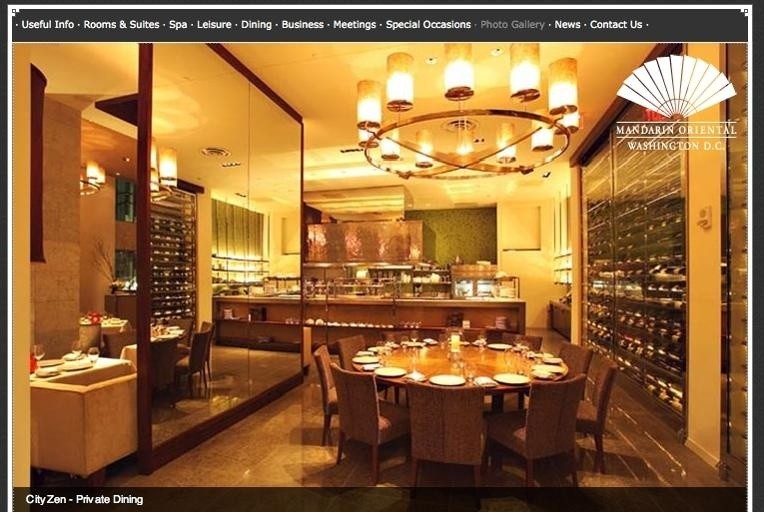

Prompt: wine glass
[
  {"left": 33, "top": 344, "right": 45, "bottom": 369},
  {"left": 72, "top": 339, "right": 99, "bottom": 366}
]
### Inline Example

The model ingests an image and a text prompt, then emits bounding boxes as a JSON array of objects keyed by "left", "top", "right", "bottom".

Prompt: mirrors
[{"left": 139, "top": 43, "right": 306, "bottom": 467}]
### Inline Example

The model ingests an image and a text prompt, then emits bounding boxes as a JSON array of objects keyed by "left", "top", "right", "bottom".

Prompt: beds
[{"left": 79, "top": 313, "right": 128, "bottom": 354}]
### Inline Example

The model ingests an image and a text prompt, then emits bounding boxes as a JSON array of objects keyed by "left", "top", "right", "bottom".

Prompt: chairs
[
  {"left": 313, "top": 322, "right": 615, "bottom": 511},
  {"left": 148, "top": 315, "right": 216, "bottom": 410},
  {"left": 29, "top": 373, "right": 137, "bottom": 478},
  {"left": 99, "top": 323, "right": 136, "bottom": 360}
]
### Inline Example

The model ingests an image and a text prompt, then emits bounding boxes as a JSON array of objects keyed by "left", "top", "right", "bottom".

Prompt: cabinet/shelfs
[
  {"left": 578, "top": 45, "right": 686, "bottom": 431},
  {"left": 552, "top": 249, "right": 570, "bottom": 286},
  {"left": 210, "top": 252, "right": 267, "bottom": 288},
  {"left": 150, "top": 188, "right": 197, "bottom": 331}
]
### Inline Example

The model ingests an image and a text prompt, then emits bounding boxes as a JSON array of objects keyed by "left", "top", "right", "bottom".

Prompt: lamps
[
  {"left": 80, "top": 158, "right": 106, "bottom": 196},
  {"left": 355, "top": 42, "right": 583, "bottom": 180},
  {"left": 150, "top": 136, "right": 178, "bottom": 196}
]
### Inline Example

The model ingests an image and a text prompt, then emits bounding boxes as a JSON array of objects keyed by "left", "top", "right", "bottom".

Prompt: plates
[
  {"left": 493, "top": 373, "right": 529, "bottom": 384},
  {"left": 375, "top": 366, "right": 405, "bottom": 377},
  {"left": 429, "top": 374, "right": 465, "bottom": 386},
  {"left": 351, "top": 336, "right": 563, "bottom": 364},
  {"left": 531, "top": 365, "right": 565, "bottom": 374}
]
